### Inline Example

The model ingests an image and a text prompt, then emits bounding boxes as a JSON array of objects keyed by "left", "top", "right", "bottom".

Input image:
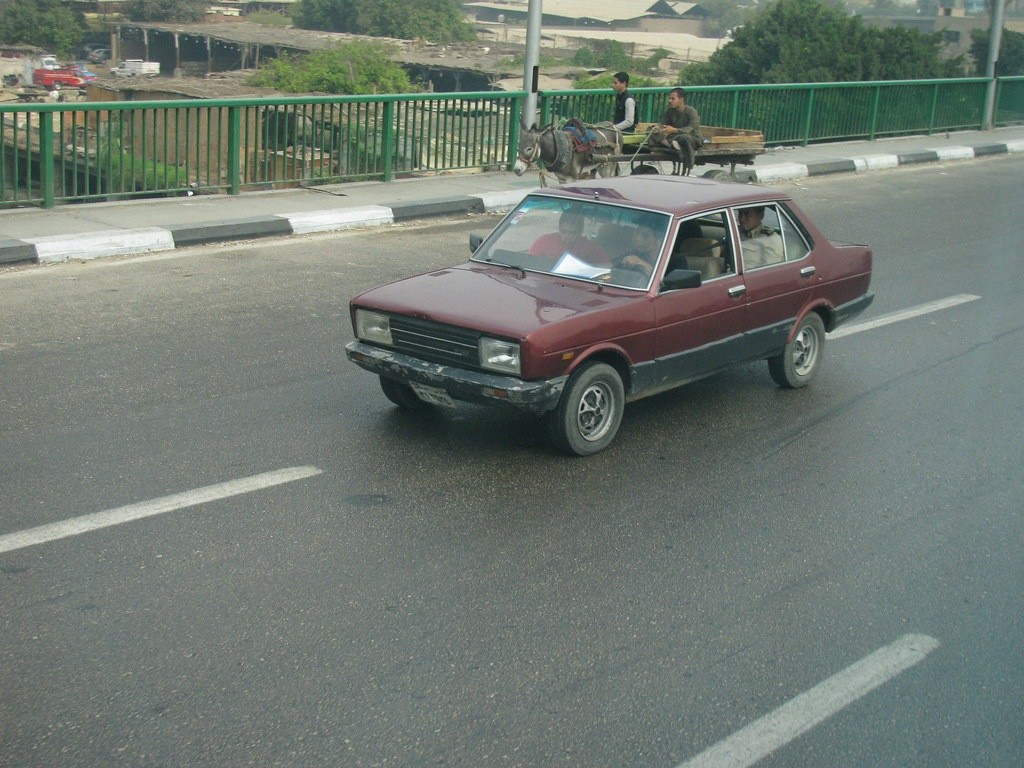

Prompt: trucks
[
  {"left": 32, "top": 62, "right": 100, "bottom": 90},
  {"left": 109, "top": 58, "right": 160, "bottom": 78}
]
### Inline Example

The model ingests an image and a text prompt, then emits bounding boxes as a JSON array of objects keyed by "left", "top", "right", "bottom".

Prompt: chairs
[
  {"left": 590, "top": 222, "right": 624, "bottom": 258},
  {"left": 680, "top": 237, "right": 725, "bottom": 280}
]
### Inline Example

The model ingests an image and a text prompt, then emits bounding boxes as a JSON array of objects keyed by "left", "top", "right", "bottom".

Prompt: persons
[
  {"left": 531, "top": 206, "right": 784, "bottom": 283},
  {"left": 652, "top": 88, "right": 703, "bottom": 167},
  {"left": 611, "top": 72, "right": 640, "bottom": 133}
]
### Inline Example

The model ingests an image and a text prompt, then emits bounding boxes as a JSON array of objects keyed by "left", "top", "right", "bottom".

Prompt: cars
[
  {"left": 38, "top": 56, "right": 61, "bottom": 70},
  {"left": 343, "top": 173, "right": 878, "bottom": 458},
  {"left": 88, "top": 48, "right": 111, "bottom": 65}
]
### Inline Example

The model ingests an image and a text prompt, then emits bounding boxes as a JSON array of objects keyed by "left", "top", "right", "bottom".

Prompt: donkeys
[{"left": 513, "top": 115, "right": 624, "bottom": 185}]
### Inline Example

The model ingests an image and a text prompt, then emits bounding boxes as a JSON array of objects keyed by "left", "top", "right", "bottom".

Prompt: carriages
[{"left": 515, "top": 117, "right": 765, "bottom": 182}]
